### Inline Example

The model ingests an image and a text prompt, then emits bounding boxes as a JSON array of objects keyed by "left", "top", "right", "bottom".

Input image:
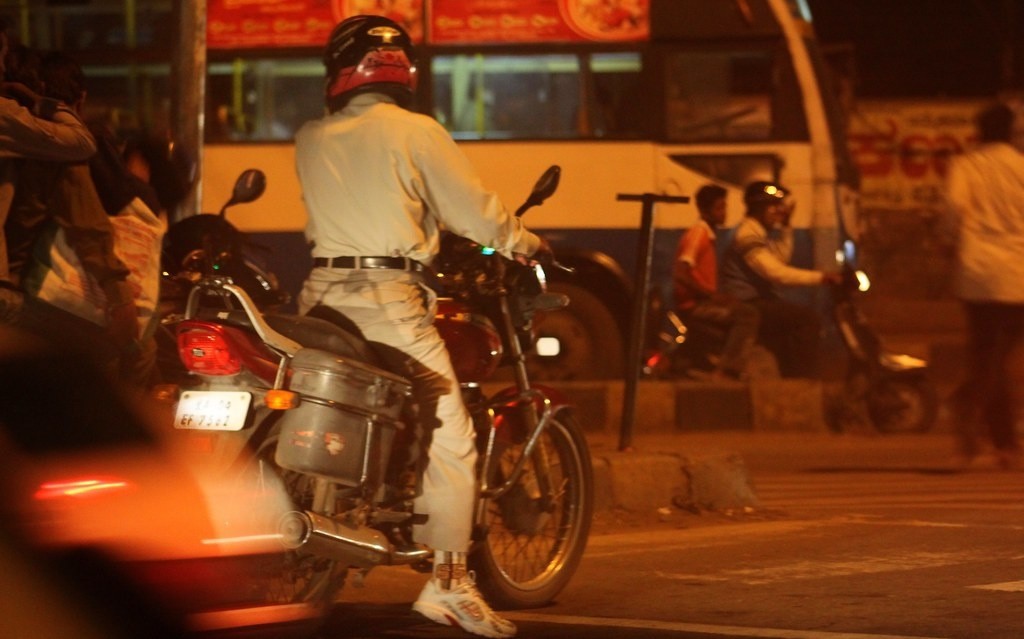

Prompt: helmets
[
  {"left": 744, "top": 182, "right": 788, "bottom": 203},
  {"left": 321, "top": 13, "right": 419, "bottom": 112}
]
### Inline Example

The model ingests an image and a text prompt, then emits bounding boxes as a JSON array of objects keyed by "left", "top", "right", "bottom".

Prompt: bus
[{"left": 0, "top": 0, "right": 868, "bottom": 380}]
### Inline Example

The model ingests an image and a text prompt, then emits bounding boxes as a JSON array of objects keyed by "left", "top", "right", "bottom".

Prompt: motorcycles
[
  {"left": 0, "top": 164, "right": 594, "bottom": 639},
  {"left": 636, "top": 230, "right": 941, "bottom": 437}
]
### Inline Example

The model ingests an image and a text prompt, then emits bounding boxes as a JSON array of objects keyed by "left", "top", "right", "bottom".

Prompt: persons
[
  {"left": 0, "top": 16, "right": 279, "bottom": 395},
  {"left": 672, "top": 184, "right": 760, "bottom": 381},
  {"left": 720, "top": 182, "right": 844, "bottom": 379},
  {"left": 933, "top": 101, "right": 1024, "bottom": 450},
  {"left": 294, "top": 14, "right": 554, "bottom": 639}
]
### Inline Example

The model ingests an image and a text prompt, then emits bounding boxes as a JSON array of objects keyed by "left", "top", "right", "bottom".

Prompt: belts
[{"left": 312, "top": 256, "right": 434, "bottom": 281}]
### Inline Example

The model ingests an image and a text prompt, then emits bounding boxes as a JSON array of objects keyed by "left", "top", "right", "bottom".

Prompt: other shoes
[{"left": 716, "top": 365, "right": 744, "bottom": 389}]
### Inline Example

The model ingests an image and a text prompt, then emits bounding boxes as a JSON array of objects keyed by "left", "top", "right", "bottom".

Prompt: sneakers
[{"left": 412, "top": 570, "right": 517, "bottom": 638}]
